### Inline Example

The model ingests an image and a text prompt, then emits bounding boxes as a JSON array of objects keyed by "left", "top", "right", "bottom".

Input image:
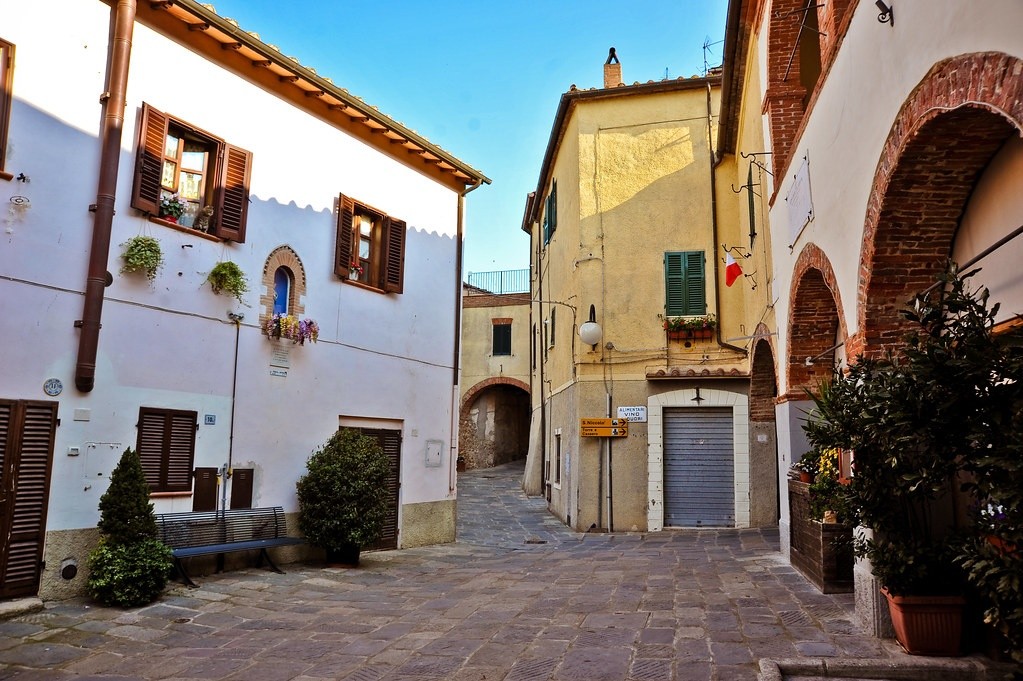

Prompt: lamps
[
  {"left": 580, "top": 304, "right": 602, "bottom": 351},
  {"left": 690, "top": 386, "right": 705, "bottom": 404},
  {"left": 875, "top": 0, "right": 894, "bottom": 27}
]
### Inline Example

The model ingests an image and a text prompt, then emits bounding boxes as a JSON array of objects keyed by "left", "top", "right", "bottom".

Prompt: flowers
[
  {"left": 265, "top": 312, "right": 319, "bottom": 346},
  {"left": 349, "top": 261, "right": 364, "bottom": 275},
  {"left": 791, "top": 458, "right": 817, "bottom": 473},
  {"left": 819, "top": 447, "right": 838, "bottom": 476},
  {"left": 160, "top": 192, "right": 190, "bottom": 219},
  {"left": 656, "top": 313, "right": 716, "bottom": 332}
]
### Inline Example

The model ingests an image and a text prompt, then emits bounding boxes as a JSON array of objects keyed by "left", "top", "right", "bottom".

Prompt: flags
[{"left": 726, "top": 252, "right": 742, "bottom": 287}]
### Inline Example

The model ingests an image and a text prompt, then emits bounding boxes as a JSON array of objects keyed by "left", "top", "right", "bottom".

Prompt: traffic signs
[{"left": 580, "top": 418, "right": 628, "bottom": 437}]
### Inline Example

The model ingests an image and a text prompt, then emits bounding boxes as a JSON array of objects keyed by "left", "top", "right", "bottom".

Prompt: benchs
[{"left": 153, "top": 505, "right": 303, "bottom": 589}]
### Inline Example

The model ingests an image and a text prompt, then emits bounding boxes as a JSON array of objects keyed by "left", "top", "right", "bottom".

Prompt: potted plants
[
  {"left": 796, "top": 260, "right": 1023, "bottom": 659},
  {"left": 296, "top": 426, "right": 392, "bottom": 565}
]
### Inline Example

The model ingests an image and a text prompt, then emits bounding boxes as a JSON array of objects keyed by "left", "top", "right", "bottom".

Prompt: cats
[{"left": 192, "top": 205, "right": 214, "bottom": 232}]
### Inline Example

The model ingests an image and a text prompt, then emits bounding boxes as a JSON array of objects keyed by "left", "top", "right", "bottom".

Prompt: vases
[
  {"left": 349, "top": 270, "right": 359, "bottom": 281},
  {"left": 160, "top": 214, "right": 177, "bottom": 223},
  {"left": 669, "top": 327, "right": 713, "bottom": 341},
  {"left": 800, "top": 471, "right": 814, "bottom": 482}
]
